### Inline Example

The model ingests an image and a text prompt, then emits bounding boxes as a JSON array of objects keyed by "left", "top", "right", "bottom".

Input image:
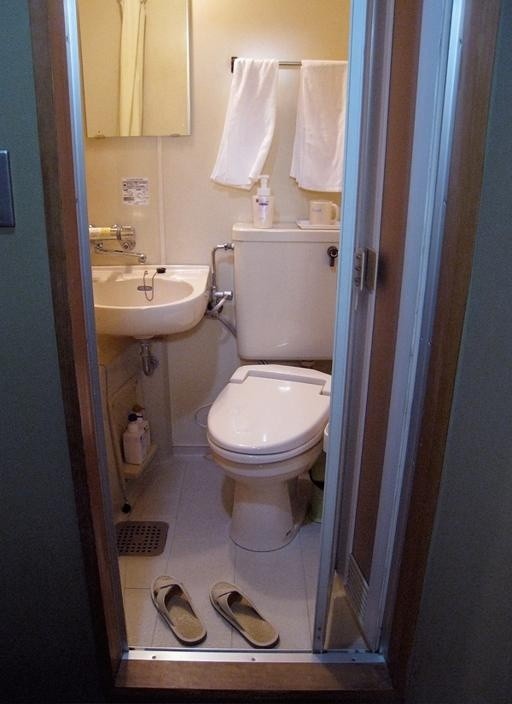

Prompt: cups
[{"left": 308, "top": 199, "right": 340, "bottom": 225}]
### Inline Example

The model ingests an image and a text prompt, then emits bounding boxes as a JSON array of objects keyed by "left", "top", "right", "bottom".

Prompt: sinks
[{"left": 91, "top": 266, "right": 212, "bottom": 339}]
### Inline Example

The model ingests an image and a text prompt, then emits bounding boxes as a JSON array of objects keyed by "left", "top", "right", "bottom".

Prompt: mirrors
[{"left": 75, "top": 1, "right": 190, "bottom": 137}]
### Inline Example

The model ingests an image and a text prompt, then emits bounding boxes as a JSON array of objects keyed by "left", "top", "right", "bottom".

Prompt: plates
[{"left": 294, "top": 218, "right": 341, "bottom": 231}]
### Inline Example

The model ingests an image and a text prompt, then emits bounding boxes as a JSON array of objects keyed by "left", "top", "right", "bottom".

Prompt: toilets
[{"left": 206, "top": 222, "right": 342, "bottom": 551}]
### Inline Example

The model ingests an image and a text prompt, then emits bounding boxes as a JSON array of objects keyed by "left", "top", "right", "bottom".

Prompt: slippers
[
  {"left": 151, "top": 575, "right": 206, "bottom": 646},
  {"left": 209, "top": 580, "right": 279, "bottom": 648}
]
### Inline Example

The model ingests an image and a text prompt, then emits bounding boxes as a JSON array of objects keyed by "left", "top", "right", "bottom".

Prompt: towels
[{"left": 211, "top": 56, "right": 347, "bottom": 192}]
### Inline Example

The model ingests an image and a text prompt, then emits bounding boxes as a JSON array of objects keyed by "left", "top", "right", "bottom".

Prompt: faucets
[{"left": 89, "top": 223, "right": 147, "bottom": 262}]
